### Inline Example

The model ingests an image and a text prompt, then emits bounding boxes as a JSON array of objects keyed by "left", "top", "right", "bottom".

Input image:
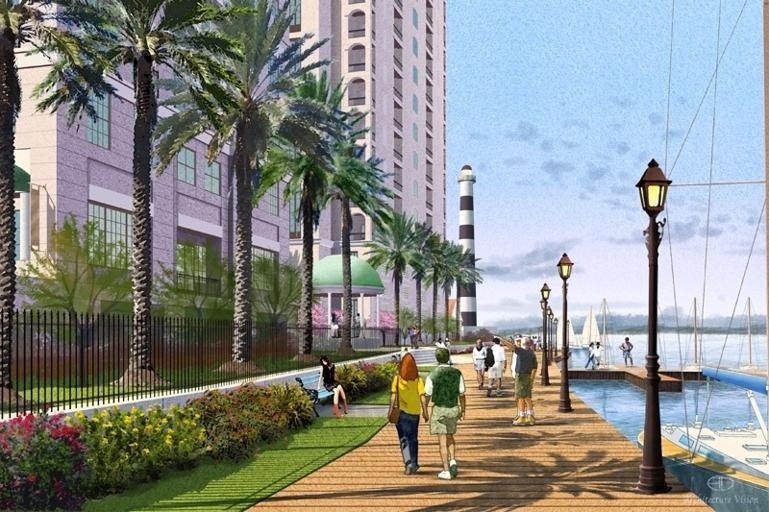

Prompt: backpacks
[{"left": 485, "top": 349, "right": 496, "bottom": 367}]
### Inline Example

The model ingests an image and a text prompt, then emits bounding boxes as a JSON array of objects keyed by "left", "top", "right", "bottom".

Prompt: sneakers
[
  {"left": 449, "top": 458, "right": 458, "bottom": 477},
  {"left": 437, "top": 470, "right": 452, "bottom": 480},
  {"left": 477, "top": 380, "right": 536, "bottom": 427}
]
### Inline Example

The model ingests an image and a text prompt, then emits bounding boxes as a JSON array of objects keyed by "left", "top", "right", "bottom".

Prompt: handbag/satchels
[{"left": 387, "top": 393, "right": 399, "bottom": 425}]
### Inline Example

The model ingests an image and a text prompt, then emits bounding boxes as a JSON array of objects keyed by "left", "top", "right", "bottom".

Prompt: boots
[
  {"left": 344, "top": 404, "right": 349, "bottom": 414},
  {"left": 332, "top": 404, "right": 342, "bottom": 417}
]
